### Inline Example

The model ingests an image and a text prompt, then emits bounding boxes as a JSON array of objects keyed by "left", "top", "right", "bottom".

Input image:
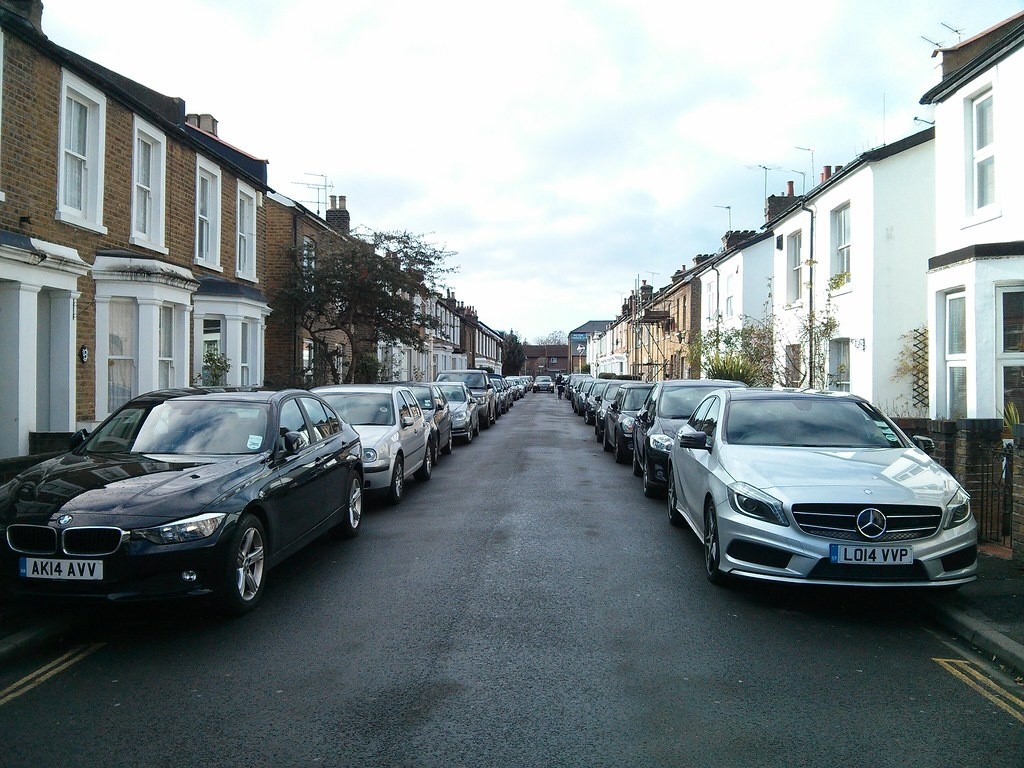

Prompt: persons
[{"left": 554, "top": 373, "right": 565, "bottom": 399}]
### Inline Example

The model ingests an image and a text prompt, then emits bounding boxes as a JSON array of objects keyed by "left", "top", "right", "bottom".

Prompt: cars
[
  {"left": 491, "top": 373, "right": 534, "bottom": 417},
  {"left": 573, "top": 379, "right": 595, "bottom": 416},
  {"left": 557, "top": 372, "right": 596, "bottom": 402},
  {"left": 427, "top": 381, "right": 479, "bottom": 443},
  {"left": 533, "top": 376, "right": 555, "bottom": 393},
  {"left": 594, "top": 381, "right": 635, "bottom": 444},
  {"left": 303, "top": 382, "right": 433, "bottom": 507},
  {"left": 584, "top": 378, "right": 612, "bottom": 425},
  {"left": 380, "top": 380, "right": 455, "bottom": 466},
  {"left": 603, "top": 380, "right": 656, "bottom": 464},
  {"left": 0, "top": 384, "right": 364, "bottom": 621},
  {"left": 632, "top": 378, "right": 750, "bottom": 498},
  {"left": 666, "top": 387, "right": 979, "bottom": 592}
]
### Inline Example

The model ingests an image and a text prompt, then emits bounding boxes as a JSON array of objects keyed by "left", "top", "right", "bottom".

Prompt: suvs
[{"left": 435, "top": 368, "right": 495, "bottom": 428}]
